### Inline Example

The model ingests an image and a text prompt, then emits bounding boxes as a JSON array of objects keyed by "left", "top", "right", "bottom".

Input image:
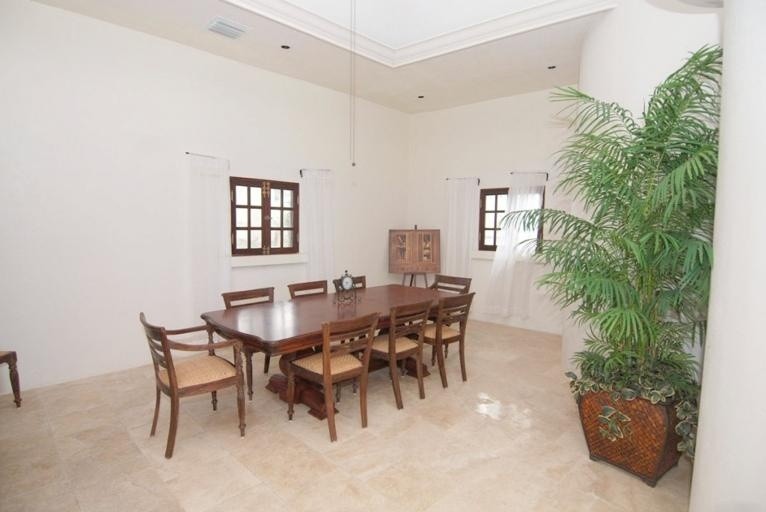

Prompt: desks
[{"left": 200, "top": 283, "right": 471, "bottom": 419}]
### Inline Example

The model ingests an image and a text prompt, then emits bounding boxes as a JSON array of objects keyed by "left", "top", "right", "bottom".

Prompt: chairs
[
  {"left": 401, "top": 291, "right": 473, "bottom": 387},
  {"left": 287, "top": 312, "right": 381, "bottom": 441},
  {"left": 354, "top": 298, "right": 434, "bottom": 410},
  {"left": 288, "top": 276, "right": 366, "bottom": 298},
  {"left": 405, "top": 276, "right": 473, "bottom": 363},
  {"left": 221, "top": 287, "right": 293, "bottom": 372},
  {"left": 140, "top": 311, "right": 247, "bottom": 458}
]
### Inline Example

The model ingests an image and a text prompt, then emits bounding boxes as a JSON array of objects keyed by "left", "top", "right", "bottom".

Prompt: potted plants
[{"left": 497, "top": 42, "right": 724, "bottom": 489}]
